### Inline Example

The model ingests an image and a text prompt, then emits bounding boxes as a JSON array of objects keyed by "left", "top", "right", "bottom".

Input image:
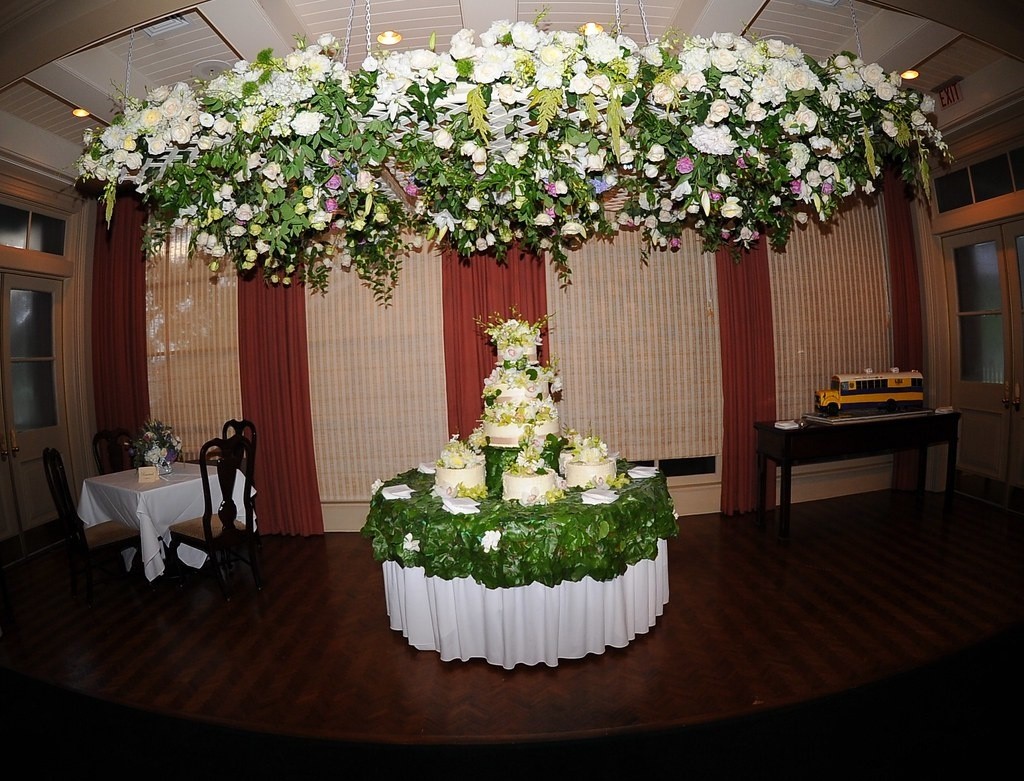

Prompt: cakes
[{"left": 435, "top": 320, "right": 617, "bottom": 504}]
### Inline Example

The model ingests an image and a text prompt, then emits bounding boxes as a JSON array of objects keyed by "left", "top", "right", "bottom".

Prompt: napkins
[
  {"left": 581, "top": 487, "right": 620, "bottom": 505},
  {"left": 381, "top": 484, "right": 417, "bottom": 500},
  {"left": 628, "top": 466, "right": 660, "bottom": 478},
  {"left": 418, "top": 461, "right": 437, "bottom": 474},
  {"left": 441, "top": 497, "right": 481, "bottom": 515}
]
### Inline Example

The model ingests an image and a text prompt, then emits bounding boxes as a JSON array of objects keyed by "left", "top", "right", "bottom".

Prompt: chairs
[
  {"left": 92, "top": 427, "right": 136, "bottom": 475},
  {"left": 43, "top": 447, "right": 141, "bottom": 609},
  {"left": 168, "top": 435, "right": 263, "bottom": 602},
  {"left": 222, "top": 419, "right": 257, "bottom": 487}
]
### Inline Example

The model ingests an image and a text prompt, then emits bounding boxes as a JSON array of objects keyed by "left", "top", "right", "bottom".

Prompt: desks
[
  {"left": 76, "top": 462, "right": 257, "bottom": 598},
  {"left": 753, "top": 408, "right": 962, "bottom": 542},
  {"left": 360, "top": 458, "right": 680, "bottom": 670}
]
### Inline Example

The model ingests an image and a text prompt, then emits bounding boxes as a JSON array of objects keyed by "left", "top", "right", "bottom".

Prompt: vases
[{"left": 156, "top": 458, "right": 172, "bottom": 475}]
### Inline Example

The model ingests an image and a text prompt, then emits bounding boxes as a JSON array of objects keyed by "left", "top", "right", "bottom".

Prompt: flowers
[
  {"left": 72, "top": 3, "right": 958, "bottom": 310},
  {"left": 124, "top": 416, "right": 182, "bottom": 470},
  {"left": 435, "top": 303, "right": 630, "bottom": 506}
]
